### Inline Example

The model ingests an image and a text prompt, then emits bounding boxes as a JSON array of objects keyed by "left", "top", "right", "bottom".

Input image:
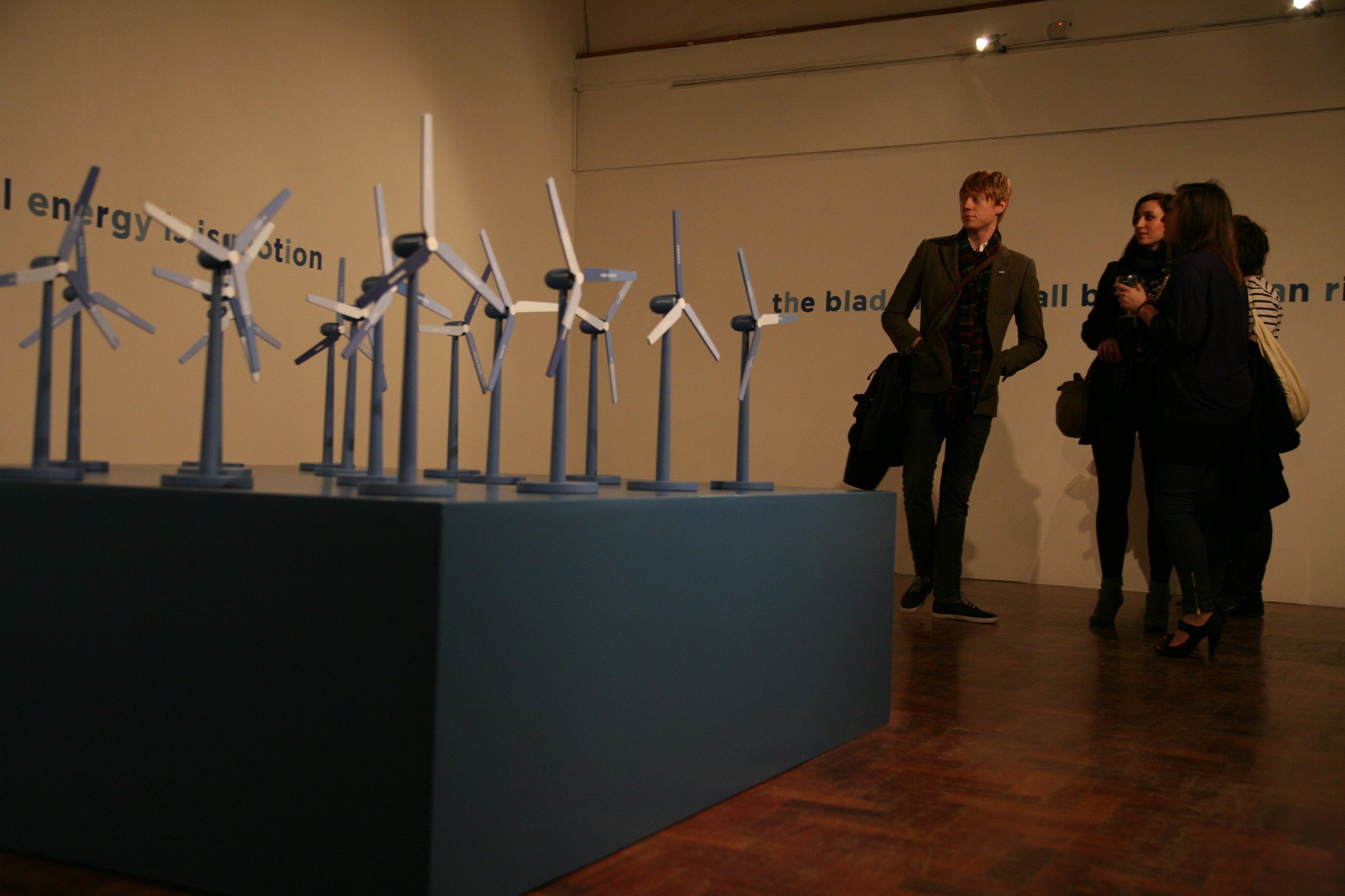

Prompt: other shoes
[{"left": 1226, "top": 595, "right": 1265, "bottom": 617}]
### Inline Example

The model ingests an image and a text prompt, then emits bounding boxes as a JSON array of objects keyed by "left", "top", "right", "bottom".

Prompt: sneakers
[
  {"left": 932, "top": 594, "right": 997, "bottom": 624},
  {"left": 899, "top": 575, "right": 933, "bottom": 613}
]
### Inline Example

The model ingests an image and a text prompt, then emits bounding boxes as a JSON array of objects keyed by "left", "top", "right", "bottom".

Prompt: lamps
[{"left": 976, "top": 33, "right": 1008, "bottom": 54}]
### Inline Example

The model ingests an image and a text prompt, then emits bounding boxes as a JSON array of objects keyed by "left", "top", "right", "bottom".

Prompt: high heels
[
  {"left": 1155, "top": 610, "right": 1223, "bottom": 657},
  {"left": 1145, "top": 591, "right": 1170, "bottom": 631},
  {"left": 1089, "top": 587, "right": 1124, "bottom": 626}
]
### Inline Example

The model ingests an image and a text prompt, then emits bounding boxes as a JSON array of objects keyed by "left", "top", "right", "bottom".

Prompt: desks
[{"left": 0, "top": 465, "right": 898, "bottom": 896}]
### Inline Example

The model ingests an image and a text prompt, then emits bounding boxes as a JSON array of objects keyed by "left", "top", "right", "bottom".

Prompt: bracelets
[{"left": 1136, "top": 301, "right": 1151, "bottom": 315}]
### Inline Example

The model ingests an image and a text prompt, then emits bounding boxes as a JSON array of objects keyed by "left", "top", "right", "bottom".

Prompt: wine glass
[{"left": 1116, "top": 274, "right": 1138, "bottom": 319}]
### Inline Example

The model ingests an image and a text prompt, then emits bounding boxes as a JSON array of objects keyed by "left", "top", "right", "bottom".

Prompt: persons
[
  {"left": 1113, "top": 183, "right": 1249, "bottom": 661},
  {"left": 1175, "top": 214, "right": 1283, "bottom": 620},
  {"left": 1081, "top": 192, "right": 1173, "bottom": 634},
  {"left": 880, "top": 169, "right": 1047, "bottom": 623}
]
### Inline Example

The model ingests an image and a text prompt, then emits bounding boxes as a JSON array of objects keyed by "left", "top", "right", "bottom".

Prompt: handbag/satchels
[
  {"left": 1055, "top": 375, "right": 1085, "bottom": 438},
  {"left": 1252, "top": 317, "right": 1310, "bottom": 425}
]
